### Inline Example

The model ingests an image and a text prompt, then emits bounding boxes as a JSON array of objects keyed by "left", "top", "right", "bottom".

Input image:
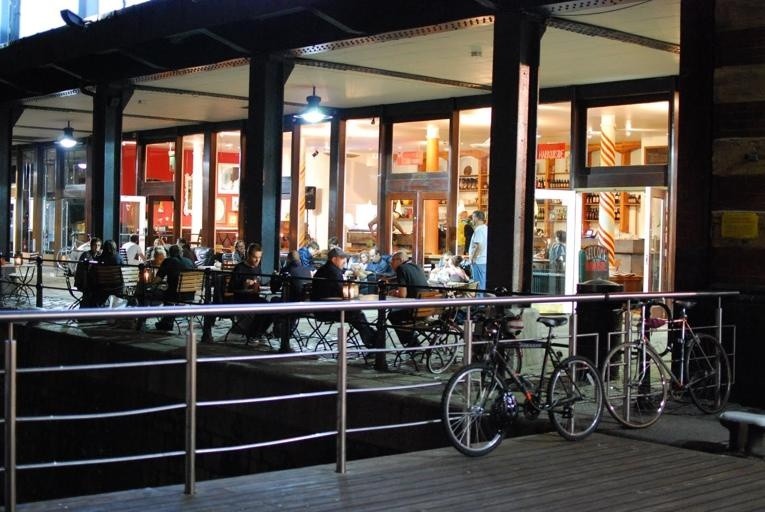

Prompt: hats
[{"left": 328, "top": 248, "right": 349, "bottom": 258}]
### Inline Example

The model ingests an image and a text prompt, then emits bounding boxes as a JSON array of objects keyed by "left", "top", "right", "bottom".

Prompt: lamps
[
  {"left": 54, "top": 119, "right": 85, "bottom": 150},
  {"left": 293, "top": 84, "right": 334, "bottom": 123}
]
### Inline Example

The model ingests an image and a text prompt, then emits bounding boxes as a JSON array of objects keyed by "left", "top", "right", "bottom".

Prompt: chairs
[{"left": 2, "top": 239, "right": 482, "bottom": 376}]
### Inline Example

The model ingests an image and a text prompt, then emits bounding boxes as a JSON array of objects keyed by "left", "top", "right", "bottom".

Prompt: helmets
[{"left": 488, "top": 388, "right": 519, "bottom": 433}]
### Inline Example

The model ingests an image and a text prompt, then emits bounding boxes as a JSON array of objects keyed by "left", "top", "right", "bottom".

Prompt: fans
[{"left": 617, "top": 120, "right": 668, "bottom": 136}]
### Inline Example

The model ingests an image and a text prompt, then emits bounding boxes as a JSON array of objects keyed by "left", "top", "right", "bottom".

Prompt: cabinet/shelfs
[{"left": 434, "top": 174, "right": 640, "bottom": 239}]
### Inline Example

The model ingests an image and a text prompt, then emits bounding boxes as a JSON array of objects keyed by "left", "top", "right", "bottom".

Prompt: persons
[
  {"left": 467, "top": 209, "right": 488, "bottom": 308},
  {"left": 73, "top": 234, "right": 198, "bottom": 331},
  {"left": 233, "top": 240, "right": 246, "bottom": 263},
  {"left": 548, "top": 230, "right": 566, "bottom": 263},
  {"left": 356, "top": 247, "right": 391, "bottom": 294},
  {"left": 384, "top": 250, "right": 430, "bottom": 359},
  {"left": 427, "top": 252, "right": 468, "bottom": 283},
  {"left": 227, "top": 243, "right": 272, "bottom": 342},
  {"left": 368, "top": 213, "right": 407, "bottom": 237},
  {"left": 270, "top": 236, "right": 376, "bottom": 358},
  {"left": 463, "top": 215, "right": 473, "bottom": 254}
]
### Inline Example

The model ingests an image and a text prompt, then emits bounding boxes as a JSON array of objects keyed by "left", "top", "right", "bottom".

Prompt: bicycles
[
  {"left": 426, "top": 302, "right": 523, "bottom": 376},
  {"left": 440, "top": 309, "right": 607, "bottom": 459},
  {"left": 55, "top": 231, "right": 92, "bottom": 272},
  {"left": 601, "top": 298, "right": 733, "bottom": 433}
]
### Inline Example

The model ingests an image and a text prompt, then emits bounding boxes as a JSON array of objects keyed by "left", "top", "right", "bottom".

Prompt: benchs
[{"left": 161, "top": 226, "right": 238, "bottom": 251}]
